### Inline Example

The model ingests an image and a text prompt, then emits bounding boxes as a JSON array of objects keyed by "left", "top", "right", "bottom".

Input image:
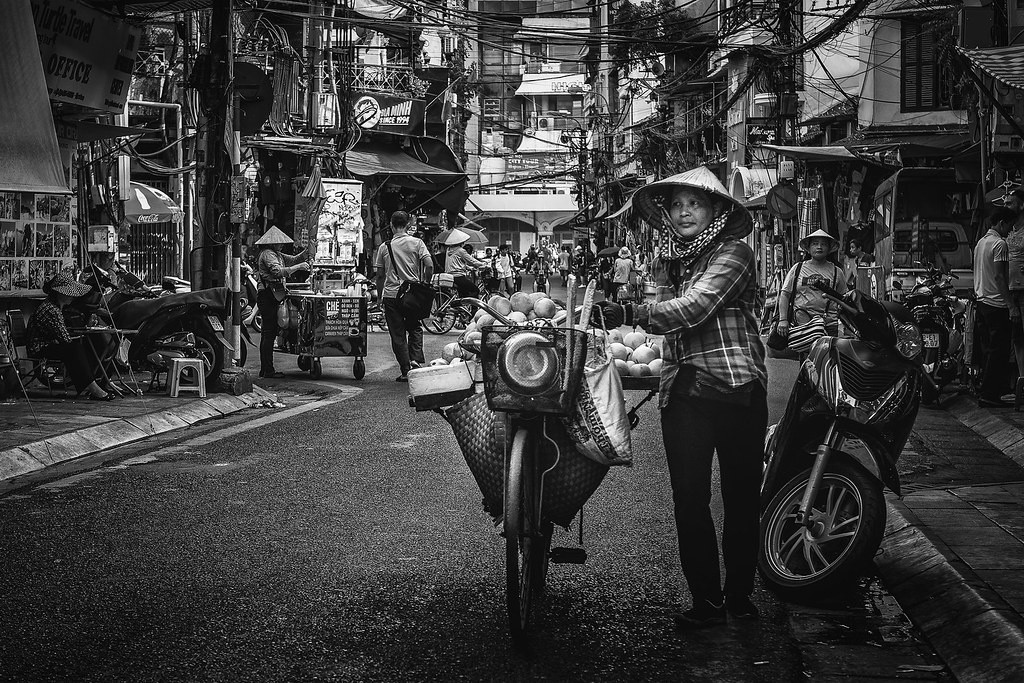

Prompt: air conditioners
[{"left": 538, "top": 115, "right": 554, "bottom": 131}]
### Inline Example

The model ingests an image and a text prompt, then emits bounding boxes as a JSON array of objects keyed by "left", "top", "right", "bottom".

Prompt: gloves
[{"left": 590, "top": 301, "right": 633, "bottom": 329}]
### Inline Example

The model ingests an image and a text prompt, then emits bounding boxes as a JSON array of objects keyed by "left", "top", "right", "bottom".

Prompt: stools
[{"left": 168, "top": 356, "right": 205, "bottom": 397}]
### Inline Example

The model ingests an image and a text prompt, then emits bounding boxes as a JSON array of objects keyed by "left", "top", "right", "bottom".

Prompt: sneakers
[{"left": 677, "top": 596, "right": 761, "bottom": 625}]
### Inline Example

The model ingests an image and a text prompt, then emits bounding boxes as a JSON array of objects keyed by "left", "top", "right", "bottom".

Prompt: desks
[{"left": 70, "top": 327, "right": 140, "bottom": 400}]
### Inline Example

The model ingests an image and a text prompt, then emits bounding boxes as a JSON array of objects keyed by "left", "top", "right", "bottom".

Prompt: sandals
[{"left": 88, "top": 384, "right": 129, "bottom": 400}]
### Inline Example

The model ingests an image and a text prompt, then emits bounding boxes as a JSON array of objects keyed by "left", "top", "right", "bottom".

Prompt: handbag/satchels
[
  {"left": 266, "top": 281, "right": 290, "bottom": 301},
  {"left": 687, "top": 367, "right": 741, "bottom": 416},
  {"left": 396, "top": 280, "right": 436, "bottom": 320},
  {"left": 767, "top": 312, "right": 789, "bottom": 350}
]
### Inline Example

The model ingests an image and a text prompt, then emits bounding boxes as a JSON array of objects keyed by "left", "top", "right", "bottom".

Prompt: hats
[
  {"left": 45, "top": 269, "right": 91, "bottom": 297},
  {"left": 632, "top": 166, "right": 753, "bottom": 239},
  {"left": 445, "top": 229, "right": 471, "bottom": 245},
  {"left": 799, "top": 229, "right": 840, "bottom": 255},
  {"left": 618, "top": 247, "right": 631, "bottom": 259},
  {"left": 255, "top": 226, "right": 294, "bottom": 244}
]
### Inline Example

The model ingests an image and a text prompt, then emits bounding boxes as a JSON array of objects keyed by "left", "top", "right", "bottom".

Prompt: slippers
[
  {"left": 396, "top": 375, "right": 407, "bottom": 382},
  {"left": 411, "top": 359, "right": 422, "bottom": 368}
]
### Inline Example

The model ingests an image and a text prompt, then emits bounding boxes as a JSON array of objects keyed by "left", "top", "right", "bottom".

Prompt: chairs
[{"left": 6, "top": 309, "right": 62, "bottom": 399}]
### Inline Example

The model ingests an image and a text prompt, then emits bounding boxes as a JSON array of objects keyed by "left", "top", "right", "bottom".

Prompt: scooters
[
  {"left": 345, "top": 272, "right": 388, "bottom": 332},
  {"left": 161, "top": 259, "right": 263, "bottom": 332}
]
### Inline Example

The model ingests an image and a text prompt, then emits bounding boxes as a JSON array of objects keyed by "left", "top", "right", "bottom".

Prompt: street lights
[
  {"left": 547, "top": 111, "right": 589, "bottom": 225},
  {"left": 568, "top": 85, "right": 616, "bottom": 249}
]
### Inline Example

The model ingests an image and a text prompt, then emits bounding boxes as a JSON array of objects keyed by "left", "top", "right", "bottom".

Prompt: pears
[
  {"left": 427, "top": 342, "right": 471, "bottom": 367},
  {"left": 588, "top": 328, "right": 665, "bottom": 378}
]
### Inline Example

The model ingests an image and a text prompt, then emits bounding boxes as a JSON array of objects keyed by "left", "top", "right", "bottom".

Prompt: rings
[{"left": 779, "top": 329, "right": 781, "bottom": 331}]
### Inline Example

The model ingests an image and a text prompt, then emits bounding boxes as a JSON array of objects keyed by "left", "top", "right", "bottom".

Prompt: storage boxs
[
  {"left": 407, "top": 360, "right": 475, "bottom": 397},
  {"left": 431, "top": 273, "right": 454, "bottom": 287}
]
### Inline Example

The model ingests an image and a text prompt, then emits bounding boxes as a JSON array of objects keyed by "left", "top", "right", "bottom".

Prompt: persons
[
  {"left": 973, "top": 190, "right": 1024, "bottom": 408},
  {"left": 254, "top": 225, "right": 310, "bottom": 378},
  {"left": 589, "top": 167, "right": 768, "bottom": 627},
  {"left": 0, "top": 190, "right": 78, "bottom": 291},
  {"left": 777, "top": 229, "right": 849, "bottom": 369},
  {"left": 376, "top": 211, "right": 434, "bottom": 381},
  {"left": 850, "top": 239, "right": 875, "bottom": 267},
  {"left": 26, "top": 272, "right": 130, "bottom": 400},
  {"left": 445, "top": 229, "right": 648, "bottom": 330}
]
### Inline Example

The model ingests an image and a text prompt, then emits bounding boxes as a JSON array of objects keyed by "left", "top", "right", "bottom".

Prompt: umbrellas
[
  {"left": 124, "top": 179, "right": 186, "bottom": 225},
  {"left": 598, "top": 247, "right": 620, "bottom": 257},
  {"left": 433, "top": 226, "right": 489, "bottom": 244}
]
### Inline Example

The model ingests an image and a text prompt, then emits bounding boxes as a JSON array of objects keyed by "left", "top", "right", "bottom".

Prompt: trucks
[{"left": 868, "top": 163, "right": 980, "bottom": 297}]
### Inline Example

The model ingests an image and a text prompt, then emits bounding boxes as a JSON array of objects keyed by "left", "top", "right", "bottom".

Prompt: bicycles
[
  {"left": 433, "top": 290, "right": 608, "bottom": 634},
  {"left": 631, "top": 275, "right": 647, "bottom": 305},
  {"left": 420, "top": 263, "right": 507, "bottom": 335}
]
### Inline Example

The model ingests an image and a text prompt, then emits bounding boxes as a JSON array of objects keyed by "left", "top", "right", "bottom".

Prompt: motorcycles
[
  {"left": 893, "top": 258, "right": 968, "bottom": 396},
  {"left": 35, "top": 259, "right": 259, "bottom": 387},
  {"left": 531, "top": 268, "right": 553, "bottom": 298},
  {"left": 760, "top": 271, "right": 926, "bottom": 597}
]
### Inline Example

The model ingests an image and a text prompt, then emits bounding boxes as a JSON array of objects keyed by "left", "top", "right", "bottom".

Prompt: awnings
[
  {"left": 344, "top": 130, "right": 470, "bottom": 234},
  {"left": 517, "top": 131, "right": 593, "bottom": 152},
  {"left": 515, "top": 74, "right": 587, "bottom": 95},
  {"left": 463, "top": 193, "right": 582, "bottom": 211},
  {"left": 955, "top": 45, "right": 1024, "bottom": 141},
  {"left": 761, "top": 143, "right": 980, "bottom": 184}
]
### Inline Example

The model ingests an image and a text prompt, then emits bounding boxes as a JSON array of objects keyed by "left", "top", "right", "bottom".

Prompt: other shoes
[
  {"left": 259, "top": 370, "right": 285, "bottom": 378},
  {"left": 979, "top": 393, "right": 1014, "bottom": 407}
]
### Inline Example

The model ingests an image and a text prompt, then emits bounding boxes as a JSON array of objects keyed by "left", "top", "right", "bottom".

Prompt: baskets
[{"left": 482, "top": 325, "right": 589, "bottom": 415}]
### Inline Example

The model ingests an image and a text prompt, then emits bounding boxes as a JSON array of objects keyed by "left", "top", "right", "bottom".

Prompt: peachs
[{"left": 464, "top": 291, "right": 567, "bottom": 344}]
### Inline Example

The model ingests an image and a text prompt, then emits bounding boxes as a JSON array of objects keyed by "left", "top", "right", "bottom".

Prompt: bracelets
[{"left": 779, "top": 319, "right": 787, "bottom": 321}]
